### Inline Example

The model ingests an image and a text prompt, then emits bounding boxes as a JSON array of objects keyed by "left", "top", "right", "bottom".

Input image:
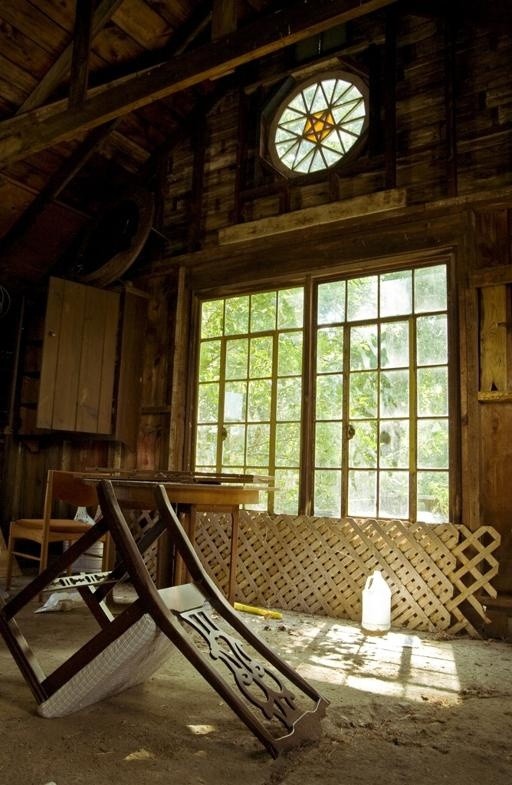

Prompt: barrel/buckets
[
  {"left": 360, "top": 567, "right": 394, "bottom": 633},
  {"left": 63, "top": 539, "right": 84, "bottom": 571},
  {"left": 80, "top": 539, "right": 104, "bottom": 572}
]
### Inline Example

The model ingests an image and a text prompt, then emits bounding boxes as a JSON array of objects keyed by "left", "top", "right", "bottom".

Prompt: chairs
[
  {"left": 4, "top": 470, "right": 119, "bottom": 602},
  {"left": 1, "top": 481, "right": 333, "bottom": 763}
]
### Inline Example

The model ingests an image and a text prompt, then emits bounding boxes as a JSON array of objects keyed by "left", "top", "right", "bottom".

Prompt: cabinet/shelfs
[{"left": 0, "top": 275, "right": 151, "bottom": 452}]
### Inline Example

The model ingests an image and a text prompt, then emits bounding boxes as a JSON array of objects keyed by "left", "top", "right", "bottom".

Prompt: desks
[{"left": 112, "top": 470, "right": 283, "bottom": 614}]
